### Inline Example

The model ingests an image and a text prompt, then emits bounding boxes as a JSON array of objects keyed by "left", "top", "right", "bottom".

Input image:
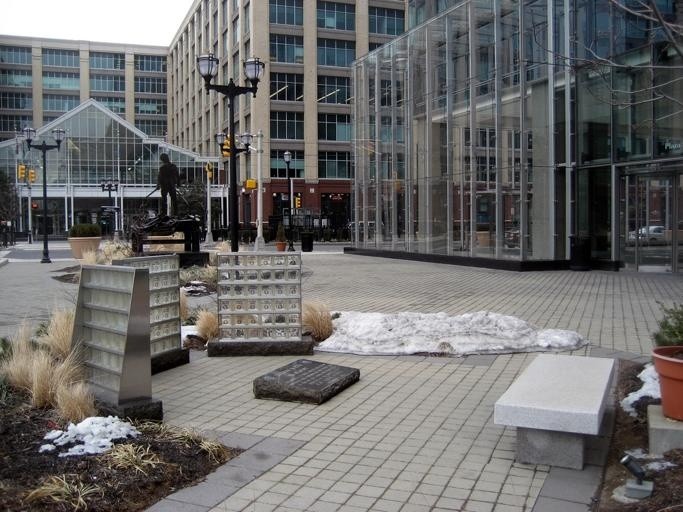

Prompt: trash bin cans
[{"left": 300, "top": 232, "right": 314, "bottom": 252}]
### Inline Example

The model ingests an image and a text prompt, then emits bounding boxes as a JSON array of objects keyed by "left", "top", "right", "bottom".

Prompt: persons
[
  {"left": 0, "top": 221, "right": 9, "bottom": 248},
  {"left": 156, "top": 153, "right": 180, "bottom": 217}
]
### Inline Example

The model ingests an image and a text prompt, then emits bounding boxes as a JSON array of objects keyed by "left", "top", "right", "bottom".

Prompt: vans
[
  {"left": 344, "top": 221, "right": 385, "bottom": 235},
  {"left": 452, "top": 231, "right": 478, "bottom": 251}
]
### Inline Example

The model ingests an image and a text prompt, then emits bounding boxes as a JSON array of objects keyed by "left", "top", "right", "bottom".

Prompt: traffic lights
[
  {"left": 295, "top": 197, "right": 301, "bottom": 208},
  {"left": 28, "top": 169, "right": 36, "bottom": 182},
  {"left": 18, "top": 164, "right": 26, "bottom": 178}
]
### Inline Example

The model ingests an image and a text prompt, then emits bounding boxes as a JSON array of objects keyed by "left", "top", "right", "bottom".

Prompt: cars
[
  {"left": 628, "top": 225, "right": 667, "bottom": 247},
  {"left": 504, "top": 229, "right": 520, "bottom": 248}
]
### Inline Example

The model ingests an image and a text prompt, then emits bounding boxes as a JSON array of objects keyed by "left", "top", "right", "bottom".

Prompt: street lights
[
  {"left": 283, "top": 151, "right": 292, "bottom": 241},
  {"left": 22, "top": 128, "right": 66, "bottom": 259},
  {"left": 100, "top": 177, "right": 119, "bottom": 205},
  {"left": 196, "top": 53, "right": 266, "bottom": 254}
]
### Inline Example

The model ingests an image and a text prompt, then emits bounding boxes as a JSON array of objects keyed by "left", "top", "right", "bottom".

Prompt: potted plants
[
  {"left": 67, "top": 222, "right": 102, "bottom": 260},
  {"left": 275, "top": 221, "right": 287, "bottom": 251}
]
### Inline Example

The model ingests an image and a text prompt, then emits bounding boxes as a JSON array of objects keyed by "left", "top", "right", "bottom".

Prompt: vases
[{"left": 653, "top": 344, "right": 683, "bottom": 422}]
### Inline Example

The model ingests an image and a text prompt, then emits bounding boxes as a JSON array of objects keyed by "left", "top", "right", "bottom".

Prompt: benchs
[{"left": 492, "top": 352, "right": 616, "bottom": 472}]
